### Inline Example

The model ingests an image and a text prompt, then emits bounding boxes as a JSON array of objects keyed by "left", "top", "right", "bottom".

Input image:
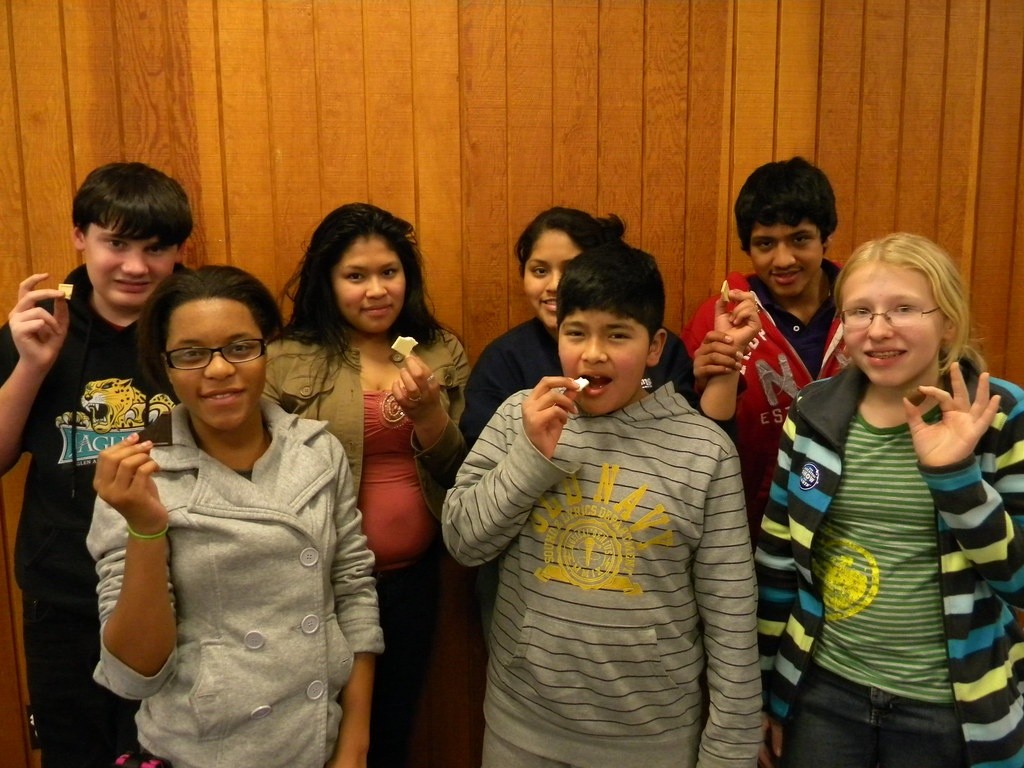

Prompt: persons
[
  {"left": 260, "top": 199, "right": 469, "bottom": 768},
  {"left": 0, "top": 161, "right": 198, "bottom": 768},
  {"left": 750, "top": 232, "right": 1024, "bottom": 768},
  {"left": 678, "top": 155, "right": 858, "bottom": 536},
  {"left": 457, "top": 206, "right": 765, "bottom": 448},
  {"left": 440, "top": 243, "right": 766, "bottom": 768},
  {"left": 83, "top": 264, "right": 386, "bottom": 768}
]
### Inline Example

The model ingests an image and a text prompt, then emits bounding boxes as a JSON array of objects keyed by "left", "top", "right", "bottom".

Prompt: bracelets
[{"left": 126, "top": 521, "right": 170, "bottom": 540}]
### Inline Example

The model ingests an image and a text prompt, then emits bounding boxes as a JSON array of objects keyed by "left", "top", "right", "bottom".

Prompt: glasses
[
  {"left": 159, "top": 335, "right": 272, "bottom": 370},
  {"left": 838, "top": 306, "right": 940, "bottom": 329}
]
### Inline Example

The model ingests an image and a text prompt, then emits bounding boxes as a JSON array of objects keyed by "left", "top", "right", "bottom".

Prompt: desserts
[
  {"left": 908, "top": 391, "right": 926, "bottom": 405},
  {"left": 721, "top": 280, "right": 730, "bottom": 302},
  {"left": 58, "top": 284, "right": 74, "bottom": 299},
  {"left": 138, "top": 411, "right": 173, "bottom": 447},
  {"left": 573, "top": 377, "right": 589, "bottom": 392},
  {"left": 392, "top": 336, "right": 418, "bottom": 356}
]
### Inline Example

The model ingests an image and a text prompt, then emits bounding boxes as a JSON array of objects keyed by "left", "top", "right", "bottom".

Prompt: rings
[
  {"left": 409, "top": 395, "right": 421, "bottom": 401},
  {"left": 427, "top": 373, "right": 434, "bottom": 381}
]
